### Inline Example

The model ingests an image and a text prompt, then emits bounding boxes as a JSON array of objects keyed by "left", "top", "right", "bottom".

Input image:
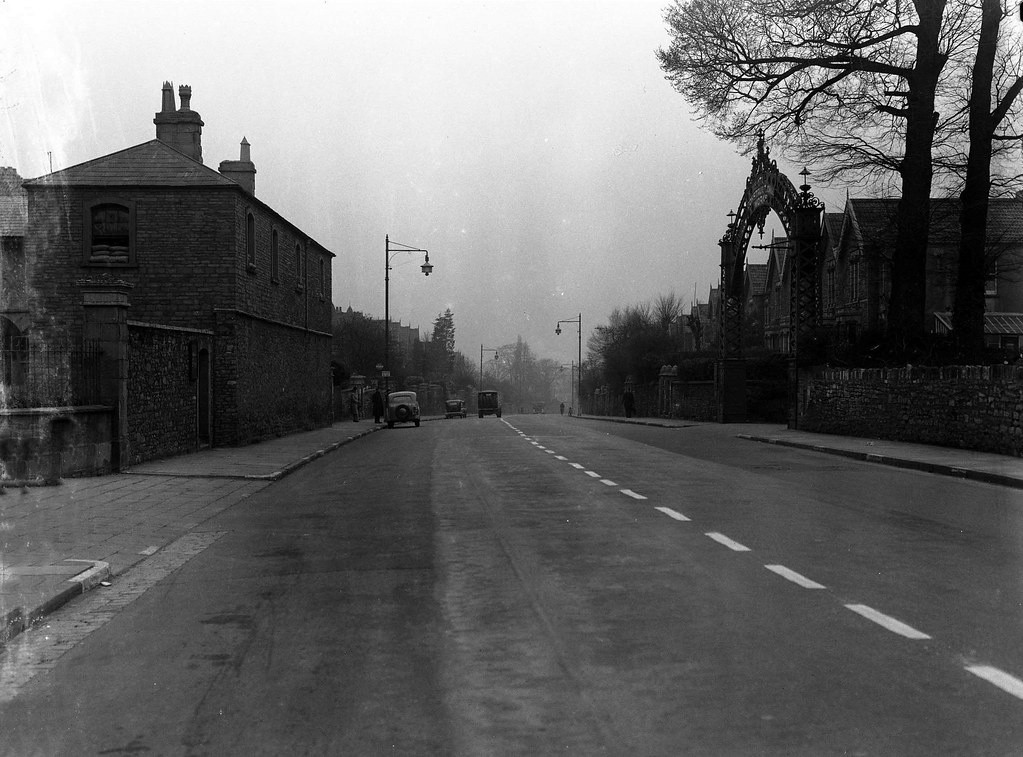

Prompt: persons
[
  {"left": 569, "top": 407, "right": 572, "bottom": 415},
  {"left": 351, "top": 387, "right": 360, "bottom": 422},
  {"left": 372, "top": 389, "right": 384, "bottom": 423},
  {"left": 560, "top": 402, "right": 564, "bottom": 414},
  {"left": 622, "top": 388, "right": 634, "bottom": 418}
]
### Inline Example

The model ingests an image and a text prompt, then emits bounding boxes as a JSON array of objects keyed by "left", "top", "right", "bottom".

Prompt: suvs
[{"left": 384, "top": 391, "right": 420, "bottom": 428}]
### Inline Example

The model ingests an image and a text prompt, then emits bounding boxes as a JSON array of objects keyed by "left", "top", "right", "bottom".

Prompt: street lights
[
  {"left": 555, "top": 313, "right": 582, "bottom": 399},
  {"left": 384, "top": 234, "right": 434, "bottom": 391},
  {"left": 560, "top": 360, "right": 574, "bottom": 408},
  {"left": 480, "top": 344, "right": 499, "bottom": 391}
]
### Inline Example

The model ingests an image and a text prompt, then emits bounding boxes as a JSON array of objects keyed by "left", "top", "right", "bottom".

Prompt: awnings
[{"left": 934, "top": 312, "right": 1023, "bottom": 334}]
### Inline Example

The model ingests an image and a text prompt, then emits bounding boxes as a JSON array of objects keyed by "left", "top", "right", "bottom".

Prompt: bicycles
[{"left": 568, "top": 407, "right": 573, "bottom": 416}]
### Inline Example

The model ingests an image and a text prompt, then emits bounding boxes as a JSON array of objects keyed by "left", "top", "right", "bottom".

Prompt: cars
[
  {"left": 533, "top": 402, "right": 546, "bottom": 414},
  {"left": 444, "top": 400, "right": 468, "bottom": 418},
  {"left": 478, "top": 390, "right": 502, "bottom": 418}
]
